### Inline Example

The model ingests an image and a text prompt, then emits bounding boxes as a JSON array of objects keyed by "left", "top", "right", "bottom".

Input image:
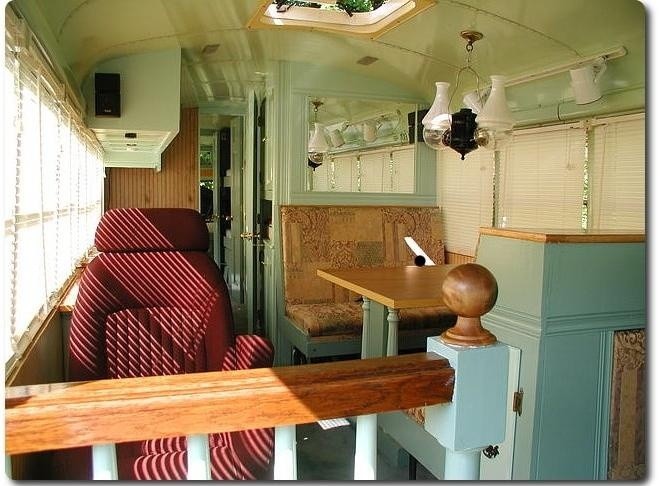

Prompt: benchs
[
  {"left": 279, "top": 203, "right": 448, "bottom": 367},
  {"left": 376, "top": 403, "right": 450, "bottom": 483}
]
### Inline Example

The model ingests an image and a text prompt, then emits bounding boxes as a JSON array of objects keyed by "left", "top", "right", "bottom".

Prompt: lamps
[
  {"left": 330, "top": 116, "right": 382, "bottom": 148},
  {"left": 421, "top": 30, "right": 514, "bottom": 160},
  {"left": 463, "top": 54, "right": 608, "bottom": 116},
  {"left": 309, "top": 99, "right": 328, "bottom": 170}
]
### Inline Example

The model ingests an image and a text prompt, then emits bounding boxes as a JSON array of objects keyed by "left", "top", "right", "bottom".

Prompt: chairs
[{"left": 64, "top": 207, "right": 277, "bottom": 481}]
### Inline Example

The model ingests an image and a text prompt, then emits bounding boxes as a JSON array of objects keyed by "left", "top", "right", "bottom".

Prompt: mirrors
[{"left": 305, "top": 95, "right": 416, "bottom": 198}]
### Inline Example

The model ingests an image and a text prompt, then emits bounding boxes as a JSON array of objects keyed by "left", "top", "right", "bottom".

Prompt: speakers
[{"left": 93, "top": 71, "right": 122, "bottom": 119}]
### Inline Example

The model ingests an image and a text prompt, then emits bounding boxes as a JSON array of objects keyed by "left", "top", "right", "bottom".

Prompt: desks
[{"left": 316, "top": 264, "right": 460, "bottom": 359}]
